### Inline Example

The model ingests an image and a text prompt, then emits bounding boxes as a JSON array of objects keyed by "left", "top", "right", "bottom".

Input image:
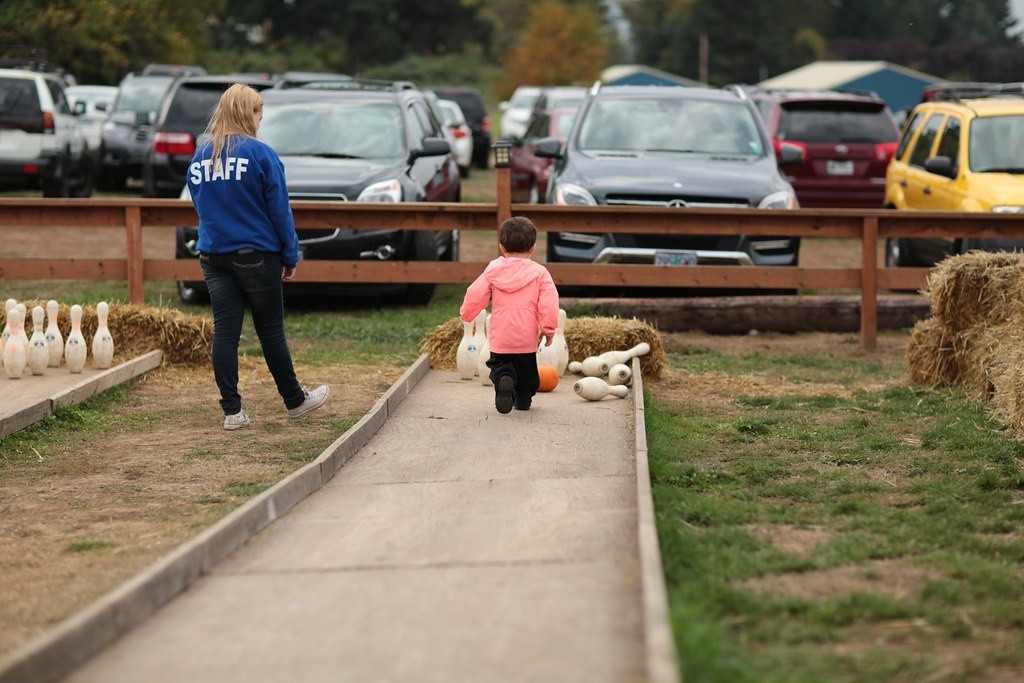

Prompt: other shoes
[
  {"left": 515, "top": 404, "right": 531, "bottom": 410},
  {"left": 495, "top": 373, "right": 515, "bottom": 415}
]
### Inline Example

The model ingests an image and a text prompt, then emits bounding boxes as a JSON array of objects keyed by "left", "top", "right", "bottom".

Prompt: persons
[
  {"left": 186, "top": 83, "right": 330, "bottom": 430},
  {"left": 460, "top": 216, "right": 559, "bottom": 415}
]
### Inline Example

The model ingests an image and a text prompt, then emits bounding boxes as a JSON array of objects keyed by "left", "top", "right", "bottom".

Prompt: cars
[
  {"left": 877, "top": 95, "right": 1024, "bottom": 292},
  {"left": 496, "top": 79, "right": 1024, "bottom": 204},
  {"left": 145, "top": 69, "right": 277, "bottom": 198},
  {"left": 527, "top": 79, "right": 801, "bottom": 295},
  {"left": 174, "top": 88, "right": 462, "bottom": 306},
  {"left": 745, "top": 87, "right": 902, "bottom": 207},
  {"left": 65, "top": 62, "right": 493, "bottom": 191},
  {"left": 0, "top": 69, "right": 95, "bottom": 198}
]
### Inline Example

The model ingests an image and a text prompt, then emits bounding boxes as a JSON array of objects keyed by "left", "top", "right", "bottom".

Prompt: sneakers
[
  {"left": 287, "top": 385, "right": 329, "bottom": 420},
  {"left": 224, "top": 408, "right": 250, "bottom": 430}
]
